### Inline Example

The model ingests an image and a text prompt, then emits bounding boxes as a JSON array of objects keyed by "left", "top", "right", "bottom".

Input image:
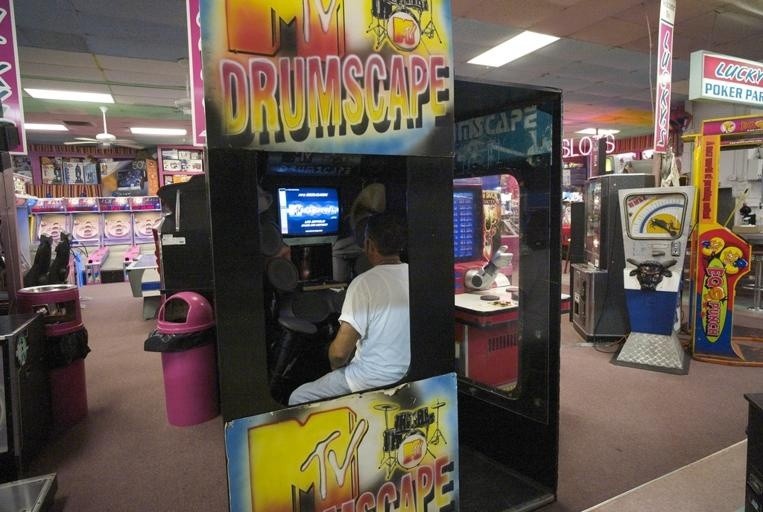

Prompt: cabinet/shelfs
[{"left": 157, "top": 145, "right": 207, "bottom": 188}]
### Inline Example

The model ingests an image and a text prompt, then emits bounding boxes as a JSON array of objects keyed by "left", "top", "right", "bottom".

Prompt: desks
[
  {"left": 742, "top": 392, "right": 762, "bottom": 512},
  {"left": 0, "top": 313, "right": 55, "bottom": 482}
]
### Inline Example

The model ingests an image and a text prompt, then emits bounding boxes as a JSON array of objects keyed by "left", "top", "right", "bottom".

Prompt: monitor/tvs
[
  {"left": 453, "top": 189, "right": 478, "bottom": 260},
  {"left": 277, "top": 184, "right": 345, "bottom": 248}
]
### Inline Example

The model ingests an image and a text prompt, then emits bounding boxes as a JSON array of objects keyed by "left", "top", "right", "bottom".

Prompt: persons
[{"left": 288, "top": 212, "right": 411, "bottom": 408}]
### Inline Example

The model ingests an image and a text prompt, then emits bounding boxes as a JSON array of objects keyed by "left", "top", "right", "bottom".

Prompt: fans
[{"left": 61, "top": 107, "right": 147, "bottom": 150}]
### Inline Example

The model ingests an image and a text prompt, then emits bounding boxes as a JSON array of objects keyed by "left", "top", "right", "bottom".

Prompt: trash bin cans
[
  {"left": 16, "top": 284, "right": 89, "bottom": 429},
  {"left": 156, "top": 291, "right": 218, "bottom": 426}
]
